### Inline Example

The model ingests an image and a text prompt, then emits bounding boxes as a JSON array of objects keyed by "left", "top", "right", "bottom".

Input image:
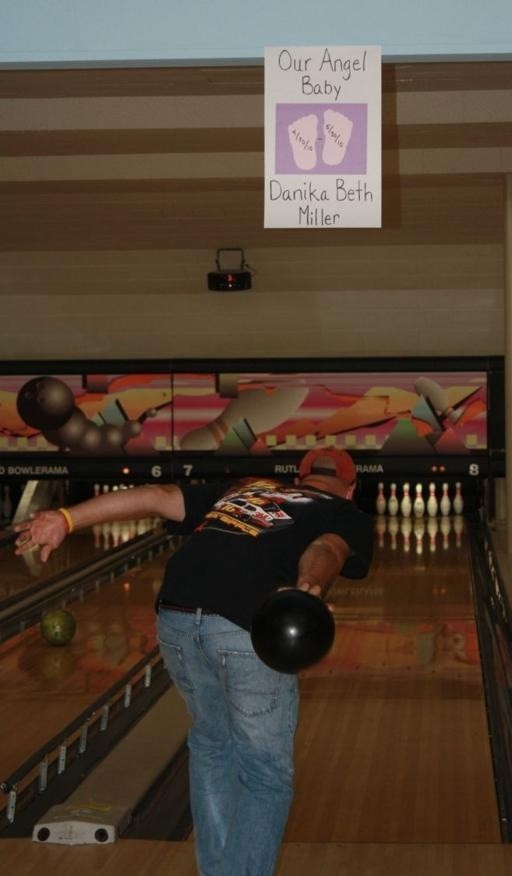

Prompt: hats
[{"left": 297, "top": 444, "right": 358, "bottom": 483}]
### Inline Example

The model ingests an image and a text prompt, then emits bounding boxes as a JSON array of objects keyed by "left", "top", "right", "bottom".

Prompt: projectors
[{"left": 206, "top": 269, "right": 251, "bottom": 292}]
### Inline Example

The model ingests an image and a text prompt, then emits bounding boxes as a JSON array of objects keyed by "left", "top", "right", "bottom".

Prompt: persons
[{"left": 12, "top": 446, "right": 378, "bottom": 875}]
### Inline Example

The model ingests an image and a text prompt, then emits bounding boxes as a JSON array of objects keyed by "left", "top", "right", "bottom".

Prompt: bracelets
[{"left": 57, "top": 507, "right": 73, "bottom": 535}]
